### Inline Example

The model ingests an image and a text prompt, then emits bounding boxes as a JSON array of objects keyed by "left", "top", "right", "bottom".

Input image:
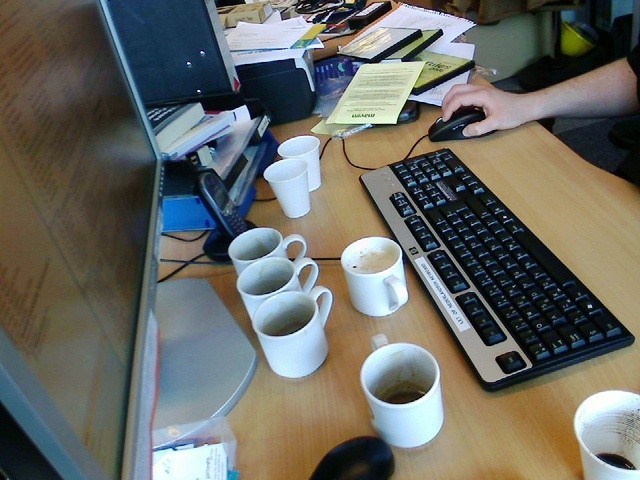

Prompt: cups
[
  {"left": 277, "top": 136, "right": 321, "bottom": 192},
  {"left": 341, "top": 236, "right": 408, "bottom": 316},
  {"left": 359, "top": 329, "right": 447, "bottom": 450},
  {"left": 235, "top": 257, "right": 320, "bottom": 333},
  {"left": 265, "top": 157, "right": 312, "bottom": 218},
  {"left": 251, "top": 285, "right": 334, "bottom": 379},
  {"left": 567, "top": 390, "right": 640, "bottom": 480},
  {"left": 226, "top": 227, "right": 307, "bottom": 285}
]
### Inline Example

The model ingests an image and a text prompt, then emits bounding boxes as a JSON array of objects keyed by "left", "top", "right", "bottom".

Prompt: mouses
[
  {"left": 309, "top": 436, "right": 394, "bottom": 480},
  {"left": 427, "top": 108, "right": 498, "bottom": 142}
]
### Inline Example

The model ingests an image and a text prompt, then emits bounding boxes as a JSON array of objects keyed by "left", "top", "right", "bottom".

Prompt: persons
[{"left": 441, "top": 43, "right": 639, "bottom": 137}]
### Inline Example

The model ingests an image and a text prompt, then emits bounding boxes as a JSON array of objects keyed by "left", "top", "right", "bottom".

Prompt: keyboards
[{"left": 358, "top": 147, "right": 635, "bottom": 393}]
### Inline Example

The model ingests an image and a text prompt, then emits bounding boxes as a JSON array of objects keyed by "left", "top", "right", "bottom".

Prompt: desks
[{"left": 152, "top": 2, "right": 639, "bottom": 478}]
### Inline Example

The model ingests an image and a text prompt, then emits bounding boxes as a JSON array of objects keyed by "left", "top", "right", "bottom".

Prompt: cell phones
[{"left": 195, "top": 172, "right": 246, "bottom": 240}]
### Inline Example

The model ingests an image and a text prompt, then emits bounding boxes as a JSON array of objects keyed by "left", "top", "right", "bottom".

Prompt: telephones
[{"left": 198, "top": 168, "right": 257, "bottom": 260}]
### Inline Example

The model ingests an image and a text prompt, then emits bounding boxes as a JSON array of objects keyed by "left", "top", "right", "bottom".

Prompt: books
[
  {"left": 140, "top": 101, "right": 251, "bottom": 163},
  {"left": 336, "top": 28, "right": 423, "bottom": 64},
  {"left": 383, "top": 28, "right": 443, "bottom": 63},
  {"left": 407, "top": 49, "right": 470, "bottom": 96}
]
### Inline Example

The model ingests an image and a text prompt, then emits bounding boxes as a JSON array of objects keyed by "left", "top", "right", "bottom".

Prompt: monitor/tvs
[{"left": 1, "top": 1, "right": 257, "bottom": 480}]
